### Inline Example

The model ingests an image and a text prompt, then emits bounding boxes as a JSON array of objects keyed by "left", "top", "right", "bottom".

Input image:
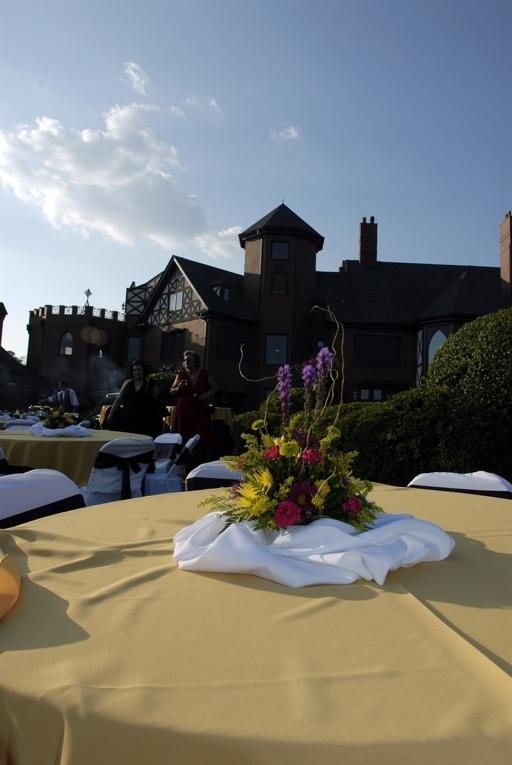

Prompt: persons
[
  {"left": 108, "top": 361, "right": 160, "bottom": 437},
  {"left": 169, "top": 350, "right": 219, "bottom": 448},
  {"left": 39, "top": 380, "right": 79, "bottom": 413}
]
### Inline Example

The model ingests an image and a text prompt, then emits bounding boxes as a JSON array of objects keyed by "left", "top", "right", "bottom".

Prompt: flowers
[
  {"left": 199, "top": 305, "right": 383, "bottom": 531},
  {"left": 35, "top": 388, "right": 77, "bottom": 428}
]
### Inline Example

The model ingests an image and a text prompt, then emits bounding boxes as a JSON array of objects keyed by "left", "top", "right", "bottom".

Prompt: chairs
[
  {"left": 0, "top": 433, "right": 246, "bottom": 528},
  {"left": 408, "top": 470, "right": 512, "bottom": 499},
  {"left": 0, "top": 410, "right": 41, "bottom": 431}
]
